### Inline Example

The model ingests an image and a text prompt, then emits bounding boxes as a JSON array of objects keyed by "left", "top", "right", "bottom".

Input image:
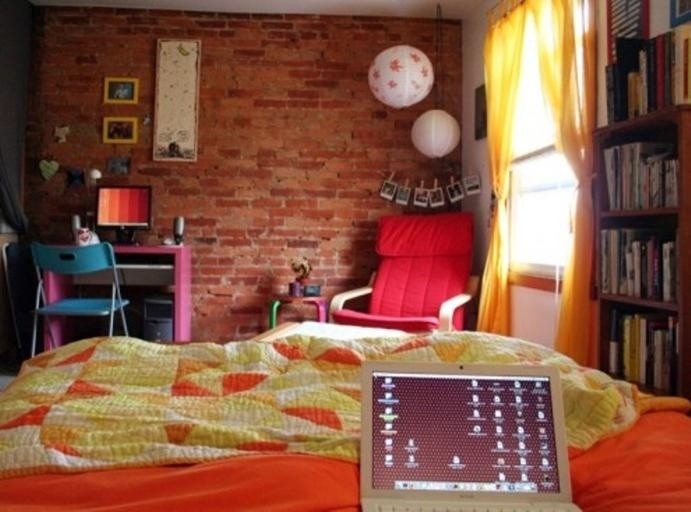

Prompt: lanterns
[
  {"left": 368, "top": 44, "right": 435, "bottom": 111},
  {"left": 412, "top": 109, "right": 462, "bottom": 160}
]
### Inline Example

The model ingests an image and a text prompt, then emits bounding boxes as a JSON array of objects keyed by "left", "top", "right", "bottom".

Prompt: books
[{"left": 597, "top": 21, "right": 690, "bottom": 396}]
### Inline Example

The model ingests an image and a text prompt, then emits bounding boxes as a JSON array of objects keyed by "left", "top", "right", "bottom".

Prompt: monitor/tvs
[{"left": 96, "top": 186, "right": 153, "bottom": 246}]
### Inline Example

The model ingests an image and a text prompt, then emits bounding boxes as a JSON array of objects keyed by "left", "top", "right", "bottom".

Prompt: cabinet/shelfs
[{"left": 590, "top": 106, "right": 690, "bottom": 397}]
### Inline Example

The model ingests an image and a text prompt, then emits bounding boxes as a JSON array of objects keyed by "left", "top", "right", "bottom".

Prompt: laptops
[{"left": 358, "top": 362, "right": 583, "bottom": 511}]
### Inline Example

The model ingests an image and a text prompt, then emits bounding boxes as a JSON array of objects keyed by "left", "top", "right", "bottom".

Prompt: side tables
[{"left": 268, "top": 294, "right": 327, "bottom": 329}]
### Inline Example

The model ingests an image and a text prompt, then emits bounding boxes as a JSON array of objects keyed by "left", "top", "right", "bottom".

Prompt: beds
[{"left": 1, "top": 337, "right": 689, "bottom": 511}]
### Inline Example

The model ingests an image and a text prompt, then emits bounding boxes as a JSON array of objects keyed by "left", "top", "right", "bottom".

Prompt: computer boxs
[{"left": 143, "top": 293, "right": 174, "bottom": 342}]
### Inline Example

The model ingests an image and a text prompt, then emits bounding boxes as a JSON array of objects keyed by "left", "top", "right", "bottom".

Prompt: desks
[{"left": 42, "top": 244, "right": 190, "bottom": 354}]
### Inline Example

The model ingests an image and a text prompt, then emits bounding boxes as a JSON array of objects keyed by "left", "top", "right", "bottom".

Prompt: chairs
[
  {"left": 29, "top": 240, "right": 131, "bottom": 362},
  {"left": 330, "top": 214, "right": 482, "bottom": 332}
]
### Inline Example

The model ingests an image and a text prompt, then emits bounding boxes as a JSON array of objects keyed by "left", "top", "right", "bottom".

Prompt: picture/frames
[
  {"left": 604, "top": 0, "right": 651, "bottom": 65},
  {"left": 103, "top": 76, "right": 139, "bottom": 143},
  {"left": 151, "top": 36, "right": 200, "bottom": 162}
]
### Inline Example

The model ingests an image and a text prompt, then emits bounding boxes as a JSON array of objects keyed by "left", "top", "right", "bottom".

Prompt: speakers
[
  {"left": 71, "top": 214, "right": 81, "bottom": 246},
  {"left": 174, "top": 217, "right": 186, "bottom": 246}
]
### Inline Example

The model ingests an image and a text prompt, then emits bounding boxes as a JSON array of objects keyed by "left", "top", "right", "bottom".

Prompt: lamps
[{"left": 369, "top": 2, "right": 463, "bottom": 158}]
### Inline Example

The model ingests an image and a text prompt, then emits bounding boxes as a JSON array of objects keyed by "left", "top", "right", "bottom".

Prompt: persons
[
  {"left": 108, "top": 122, "right": 132, "bottom": 138},
  {"left": 113, "top": 83, "right": 130, "bottom": 99}
]
